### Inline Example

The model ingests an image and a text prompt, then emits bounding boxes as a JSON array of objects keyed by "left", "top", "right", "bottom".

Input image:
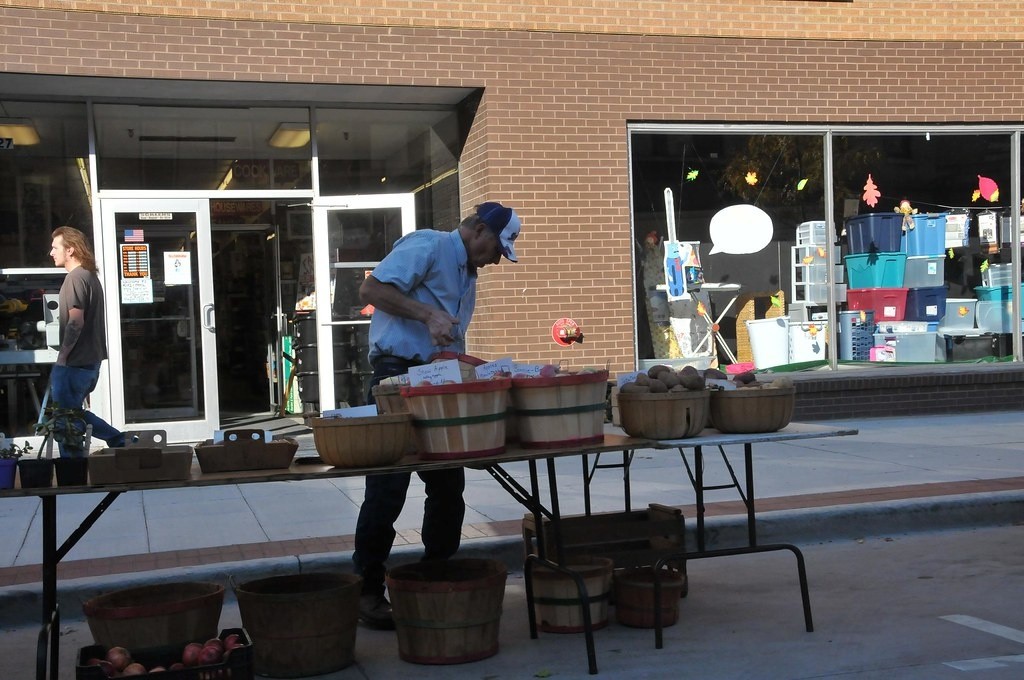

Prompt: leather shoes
[{"left": 357, "top": 588, "right": 396, "bottom": 628}]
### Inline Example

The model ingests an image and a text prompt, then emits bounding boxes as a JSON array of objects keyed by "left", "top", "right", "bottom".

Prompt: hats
[{"left": 475, "top": 203, "right": 522, "bottom": 263}]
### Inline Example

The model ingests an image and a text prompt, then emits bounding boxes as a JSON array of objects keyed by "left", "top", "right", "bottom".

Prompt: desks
[{"left": 0, "top": 425, "right": 857, "bottom": 680}]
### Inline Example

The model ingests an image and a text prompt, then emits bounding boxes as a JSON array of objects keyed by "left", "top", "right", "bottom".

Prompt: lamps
[
  {"left": 268, "top": 123, "right": 320, "bottom": 149},
  {"left": 0, "top": 119, "right": 39, "bottom": 146}
]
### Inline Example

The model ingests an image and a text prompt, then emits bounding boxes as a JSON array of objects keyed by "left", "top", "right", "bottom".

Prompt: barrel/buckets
[
  {"left": 615, "top": 566, "right": 684, "bottom": 629},
  {"left": 82, "top": 580, "right": 226, "bottom": 658},
  {"left": 385, "top": 558, "right": 507, "bottom": 665},
  {"left": 529, "top": 557, "right": 613, "bottom": 634},
  {"left": 938, "top": 299, "right": 978, "bottom": 330},
  {"left": 371, "top": 351, "right": 609, "bottom": 462},
  {"left": 231, "top": 571, "right": 361, "bottom": 679}
]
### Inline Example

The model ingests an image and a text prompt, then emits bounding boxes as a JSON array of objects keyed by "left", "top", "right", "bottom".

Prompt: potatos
[
  {"left": 620, "top": 365, "right": 707, "bottom": 393},
  {"left": 704, "top": 368, "right": 795, "bottom": 388}
]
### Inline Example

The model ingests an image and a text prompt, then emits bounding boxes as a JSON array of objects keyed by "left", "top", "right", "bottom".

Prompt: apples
[
  {"left": 414, "top": 363, "right": 597, "bottom": 387},
  {"left": 86, "top": 634, "right": 245, "bottom": 680}
]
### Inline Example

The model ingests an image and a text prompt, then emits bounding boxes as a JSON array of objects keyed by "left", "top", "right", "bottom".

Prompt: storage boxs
[{"left": 74, "top": 212, "right": 1023, "bottom": 680}]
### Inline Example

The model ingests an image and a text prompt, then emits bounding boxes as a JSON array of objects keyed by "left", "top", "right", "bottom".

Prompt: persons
[
  {"left": 352, "top": 202, "right": 521, "bottom": 631},
  {"left": 50, "top": 226, "right": 121, "bottom": 457}
]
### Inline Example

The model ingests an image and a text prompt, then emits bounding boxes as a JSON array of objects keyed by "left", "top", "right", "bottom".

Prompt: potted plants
[{"left": 1, "top": 404, "right": 89, "bottom": 492}]
